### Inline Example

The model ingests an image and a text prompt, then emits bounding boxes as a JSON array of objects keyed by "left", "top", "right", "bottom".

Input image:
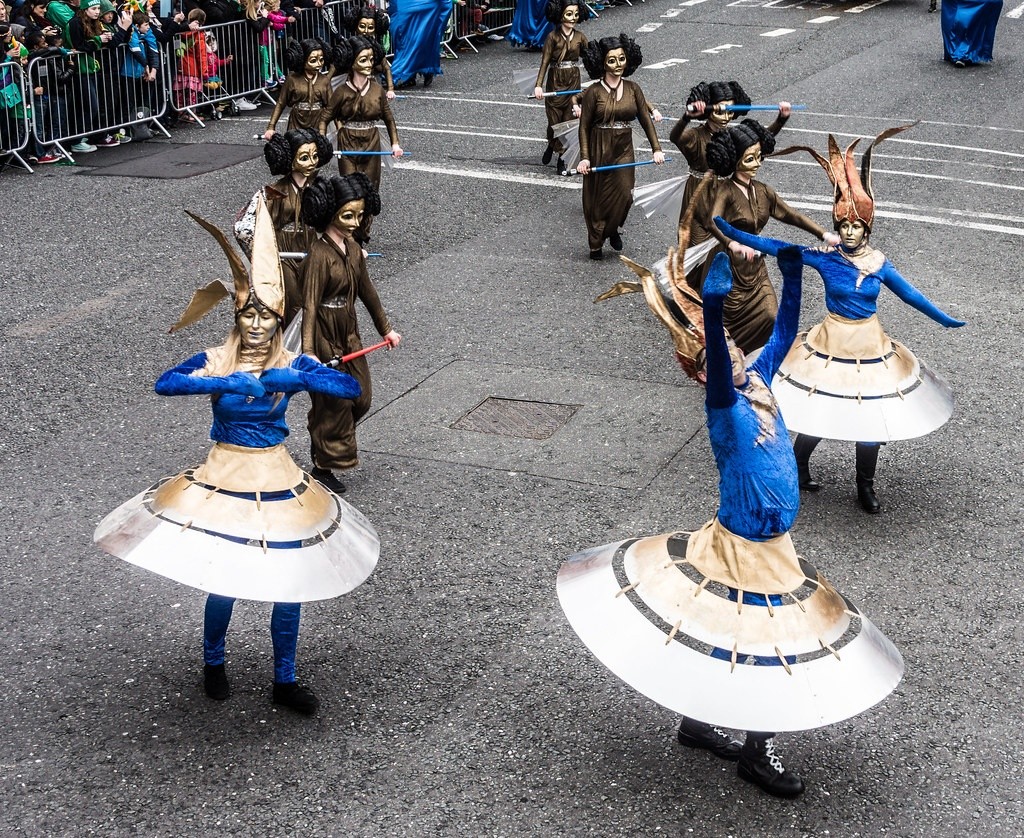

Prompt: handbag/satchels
[{"left": 0, "top": 83, "right": 21, "bottom": 109}]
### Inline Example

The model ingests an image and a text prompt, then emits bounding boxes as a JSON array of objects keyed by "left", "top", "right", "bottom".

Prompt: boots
[
  {"left": 856, "top": 441, "right": 882, "bottom": 513},
  {"left": 793, "top": 433, "right": 822, "bottom": 491}
]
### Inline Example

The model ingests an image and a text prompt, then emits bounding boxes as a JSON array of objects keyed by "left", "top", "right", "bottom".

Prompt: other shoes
[
  {"left": 311, "top": 467, "right": 346, "bottom": 493},
  {"left": 610, "top": 230, "right": 623, "bottom": 250},
  {"left": 542, "top": 142, "right": 555, "bottom": 165},
  {"left": 955, "top": 59, "right": 966, "bottom": 67},
  {"left": 404, "top": 24, "right": 505, "bottom": 86},
  {"left": 181, "top": 75, "right": 286, "bottom": 121},
  {"left": 557, "top": 152, "right": 567, "bottom": 175},
  {"left": 589, "top": 248, "right": 603, "bottom": 261},
  {"left": 927, "top": 2, "right": 937, "bottom": 13},
  {"left": 588, "top": 1, "right": 623, "bottom": 19}
]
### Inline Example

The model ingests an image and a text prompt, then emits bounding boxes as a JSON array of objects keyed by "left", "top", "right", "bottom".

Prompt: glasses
[{"left": 697, "top": 338, "right": 735, "bottom": 369}]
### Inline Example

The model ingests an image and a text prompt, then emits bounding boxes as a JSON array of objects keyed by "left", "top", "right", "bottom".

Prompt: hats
[
  {"left": 9, "top": 41, "right": 30, "bottom": 56},
  {"left": 167, "top": 191, "right": 286, "bottom": 335},
  {"left": 761, "top": 118, "right": 922, "bottom": 244},
  {"left": 591, "top": 169, "right": 731, "bottom": 380},
  {"left": 80, "top": 0, "right": 101, "bottom": 9}
]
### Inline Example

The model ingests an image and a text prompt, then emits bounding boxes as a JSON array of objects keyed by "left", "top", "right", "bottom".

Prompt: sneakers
[
  {"left": 19, "top": 127, "right": 161, "bottom": 163},
  {"left": 273, "top": 681, "right": 321, "bottom": 715},
  {"left": 739, "top": 735, "right": 806, "bottom": 800},
  {"left": 678, "top": 716, "right": 744, "bottom": 761},
  {"left": 203, "top": 663, "right": 230, "bottom": 701}
]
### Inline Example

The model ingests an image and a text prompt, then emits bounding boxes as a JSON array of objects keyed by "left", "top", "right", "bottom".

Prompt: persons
[
  {"left": 91, "top": 189, "right": 382, "bottom": 714},
  {"left": 235, "top": 128, "right": 333, "bottom": 356},
  {"left": 505, "top": 0, "right": 553, "bottom": 51},
  {"left": 713, "top": 119, "right": 967, "bottom": 512},
  {"left": 262, "top": 36, "right": 342, "bottom": 139},
  {"left": 670, "top": 79, "right": 791, "bottom": 357},
  {"left": 707, "top": 118, "right": 841, "bottom": 354},
  {"left": 578, "top": 32, "right": 666, "bottom": 259},
  {"left": 319, "top": 36, "right": 404, "bottom": 248},
  {"left": 328, "top": 5, "right": 397, "bottom": 100},
  {"left": 386, "top": 0, "right": 454, "bottom": 87},
  {"left": 301, "top": 171, "right": 401, "bottom": 494},
  {"left": 0, "top": 0, "right": 393, "bottom": 164},
  {"left": 585, "top": 0, "right": 616, "bottom": 19},
  {"left": 556, "top": 244, "right": 904, "bottom": 796},
  {"left": 439, "top": 0, "right": 517, "bottom": 59},
  {"left": 533, "top": 0, "right": 588, "bottom": 177},
  {"left": 942, "top": 0, "right": 1003, "bottom": 67}
]
